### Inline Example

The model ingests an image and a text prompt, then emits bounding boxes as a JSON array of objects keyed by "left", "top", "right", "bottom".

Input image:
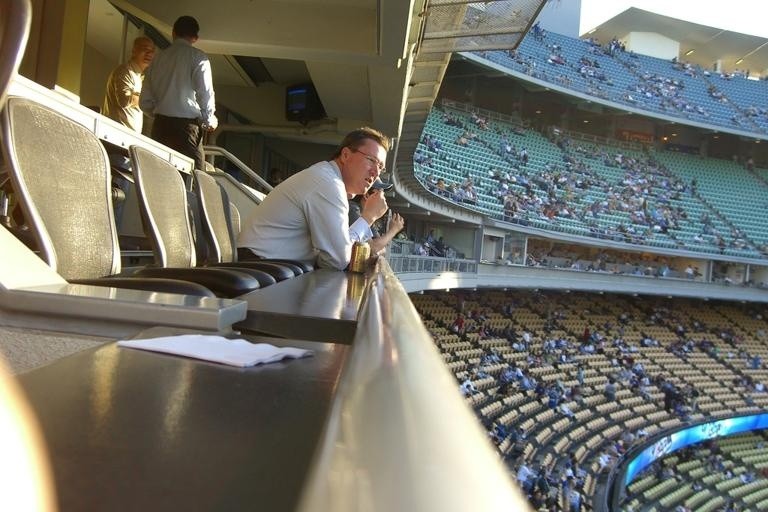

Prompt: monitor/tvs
[{"left": 285, "top": 86, "right": 321, "bottom": 120}]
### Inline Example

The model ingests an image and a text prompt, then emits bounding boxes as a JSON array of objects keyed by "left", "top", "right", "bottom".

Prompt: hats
[{"left": 370, "top": 177, "right": 394, "bottom": 192}]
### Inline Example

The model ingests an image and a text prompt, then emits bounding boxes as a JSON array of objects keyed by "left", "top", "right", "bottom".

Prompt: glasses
[{"left": 350, "top": 146, "right": 387, "bottom": 174}]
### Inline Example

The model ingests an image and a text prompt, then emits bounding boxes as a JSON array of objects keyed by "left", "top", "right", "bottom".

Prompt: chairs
[
  {"left": 421, "top": 99, "right": 768, "bottom": 270},
  {"left": 412, "top": 287, "right": 768, "bottom": 511},
  {"left": 5, "top": 93, "right": 256, "bottom": 308},
  {"left": 127, "top": 144, "right": 292, "bottom": 285},
  {"left": 188, "top": 167, "right": 313, "bottom": 277},
  {"left": 457, "top": 22, "right": 768, "bottom": 146}
]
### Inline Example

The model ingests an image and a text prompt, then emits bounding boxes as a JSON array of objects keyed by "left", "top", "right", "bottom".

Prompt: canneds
[{"left": 349, "top": 242, "right": 371, "bottom": 274}]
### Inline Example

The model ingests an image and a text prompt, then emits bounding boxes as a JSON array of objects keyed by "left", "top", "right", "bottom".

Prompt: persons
[
  {"left": 262, "top": 168, "right": 282, "bottom": 195},
  {"left": 101, "top": 37, "right": 155, "bottom": 233},
  {"left": 413, "top": 21, "right": 768, "bottom": 260},
  {"left": 399, "top": 229, "right": 768, "bottom": 511},
  {"left": 235, "top": 127, "right": 389, "bottom": 270},
  {"left": 348, "top": 177, "right": 404, "bottom": 257},
  {"left": 139, "top": 16, "right": 217, "bottom": 172}
]
